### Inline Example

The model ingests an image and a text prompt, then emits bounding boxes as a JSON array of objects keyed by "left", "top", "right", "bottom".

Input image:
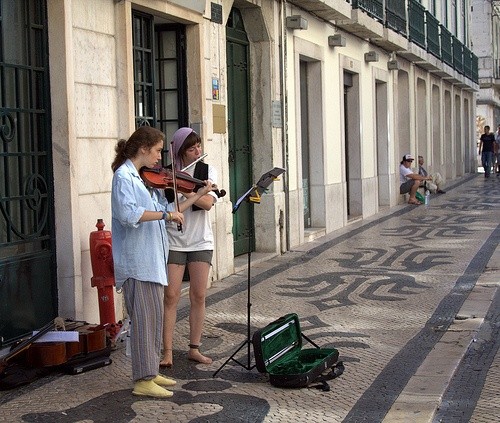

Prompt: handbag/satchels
[{"left": 415, "top": 192, "right": 424, "bottom": 201}]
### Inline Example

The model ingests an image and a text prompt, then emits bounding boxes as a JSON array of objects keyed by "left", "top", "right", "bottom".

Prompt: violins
[{"left": 139, "top": 164, "right": 226, "bottom": 198}]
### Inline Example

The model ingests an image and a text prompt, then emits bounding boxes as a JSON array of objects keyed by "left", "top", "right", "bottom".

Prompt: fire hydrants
[{"left": 88, "top": 218, "right": 123, "bottom": 345}]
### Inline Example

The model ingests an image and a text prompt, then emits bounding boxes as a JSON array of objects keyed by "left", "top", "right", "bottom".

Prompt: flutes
[{"left": 180, "top": 153, "right": 208, "bottom": 172}]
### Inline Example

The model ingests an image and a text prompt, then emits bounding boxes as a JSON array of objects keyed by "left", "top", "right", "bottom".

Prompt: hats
[
  {"left": 171, "top": 128, "right": 198, "bottom": 157},
  {"left": 403, "top": 154, "right": 414, "bottom": 161}
]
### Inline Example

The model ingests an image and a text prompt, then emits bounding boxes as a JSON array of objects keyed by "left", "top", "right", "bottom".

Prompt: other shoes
[
  {"left": 408, "top": 200, "right": 423, "bottom": 205},
  {"left": 485, "top": 172, "right": 490, "bottom": 177},
  {"left": 437, "top": 190, "right": 446, "bottom": 194},
  {"left": 496, "top": 172, "right": 500, "bottom": 174}
]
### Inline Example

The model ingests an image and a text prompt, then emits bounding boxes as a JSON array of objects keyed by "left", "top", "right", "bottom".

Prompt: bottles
[
  {"left": 125, "top": 320, "right": 132, "bottom": 357},
  {"left": 425, "top": 189, "right": 431, "bottom": 205}
]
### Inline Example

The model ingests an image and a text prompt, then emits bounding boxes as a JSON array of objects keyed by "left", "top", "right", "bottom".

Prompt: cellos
[{"left": 0, "top": 319, "right": 110, "bottom": 374}]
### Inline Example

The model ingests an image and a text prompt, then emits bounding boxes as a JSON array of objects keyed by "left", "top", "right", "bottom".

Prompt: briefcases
[{"left": 253, "top": 313, "right": 339, "bottom": 388}]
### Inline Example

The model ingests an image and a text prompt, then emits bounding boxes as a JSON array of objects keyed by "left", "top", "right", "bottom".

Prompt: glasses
[{"left": 407, "top": 160, "right": 412, "bottom": 162}]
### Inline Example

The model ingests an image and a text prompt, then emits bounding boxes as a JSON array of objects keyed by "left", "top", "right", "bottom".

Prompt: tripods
[{"left": 211, "top": 167, "right": 287, "bottom": 378}]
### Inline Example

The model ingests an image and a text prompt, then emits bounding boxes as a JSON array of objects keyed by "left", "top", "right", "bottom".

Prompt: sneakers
[
  {"left": 153, "top": 375, "right": 176, "bottom": 385},
  {"left": 132, "top": 380, "right": 174, "bottom": 396}
]
